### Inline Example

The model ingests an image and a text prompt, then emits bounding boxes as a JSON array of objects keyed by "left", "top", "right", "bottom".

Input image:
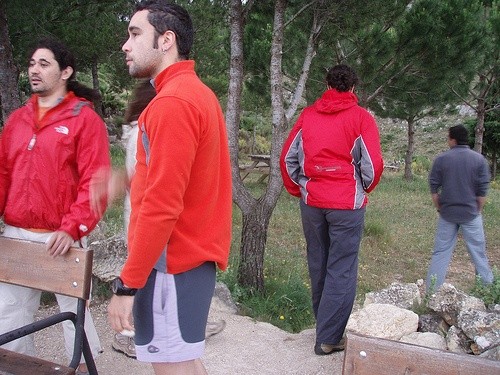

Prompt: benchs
[
  {"left": 239, "top": 165, "right": 269, "bottom": 171},
  {"left": 342, "top": 332, "right": 500, "bottom": 375},
  {"left": 0, "top": 236, "right": 99, "bottom": 375}
]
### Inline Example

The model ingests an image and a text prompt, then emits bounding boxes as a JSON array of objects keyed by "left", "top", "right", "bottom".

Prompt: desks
[{"left": 241, "top": 155, "right": 270, "bottom": 183}]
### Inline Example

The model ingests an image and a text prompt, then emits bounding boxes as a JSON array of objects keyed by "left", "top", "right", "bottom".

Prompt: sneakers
[
  {"left": 112, "top": 332, "right": 136, "bottom": 358},
  {"left": 315, "top": 336, "right": 347, "bottom": 355},
  {"left": 205, "top": 320, "right": 226, "bottom": 339}
]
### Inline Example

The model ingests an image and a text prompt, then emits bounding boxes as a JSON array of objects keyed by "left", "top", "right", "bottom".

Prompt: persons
[
  {"left": 106, "top": 1, "right": 232, "bottom": 375},
  {"left": 279, "top": 64, "right": 384, "bottom": 355},
  {"left": 0, "top": 42, "right": 111, "bottom": 375},
  {"left": 112, "top": 78, "right": 226, "bottom": 360},
  {"left": 424, "top": 126, "right": 494, "bottom": 295}
]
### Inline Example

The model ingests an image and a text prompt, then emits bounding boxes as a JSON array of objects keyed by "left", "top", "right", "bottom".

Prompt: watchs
[{"left": 111, "top": 277, "right": 138, "bottom": 296}]
